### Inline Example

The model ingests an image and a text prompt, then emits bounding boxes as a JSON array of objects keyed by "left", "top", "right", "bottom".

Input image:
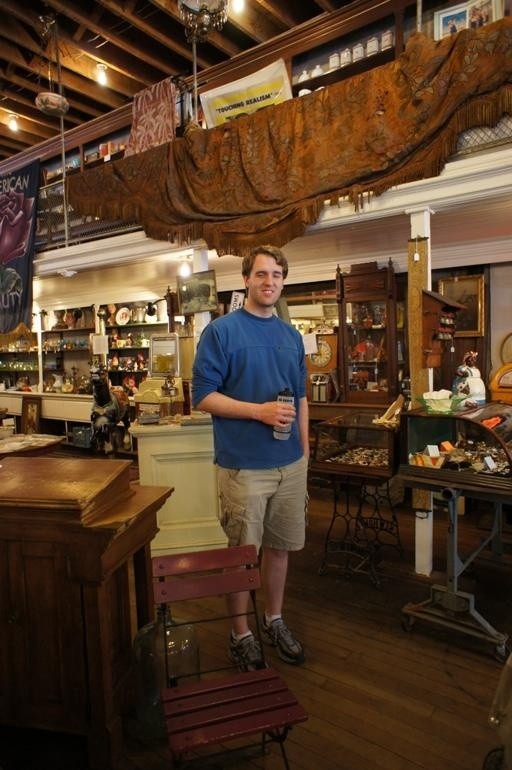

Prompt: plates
[
  {"left": 415, "top": 397, "right": 469, "bottom": 415},
  {"left": 115, "top": 307, "right": 131, "bottom": 327}
]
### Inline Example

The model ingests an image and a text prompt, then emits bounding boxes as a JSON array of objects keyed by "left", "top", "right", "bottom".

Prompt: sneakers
[
  {"left": 227, "top": 631, "right": 269, "bottom": 672},
  {"left": 261, "top": 618, "right": 306, "bottom": 664}
]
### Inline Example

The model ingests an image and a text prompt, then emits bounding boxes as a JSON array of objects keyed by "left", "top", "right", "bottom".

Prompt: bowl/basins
[{"left": 1, "top": 426, "right": 15, "bottom": 440}]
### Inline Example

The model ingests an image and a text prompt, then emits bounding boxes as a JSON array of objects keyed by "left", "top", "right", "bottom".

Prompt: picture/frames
[
  {"left": 437, "top": 273, "right": 485, "bottom": 339},
  {"left": 433, "top": 0, "right": 502, "bottom": 42}
]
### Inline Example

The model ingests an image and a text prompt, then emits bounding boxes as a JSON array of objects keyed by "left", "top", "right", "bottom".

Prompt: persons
[
  {"left": 190, "top": 244, "right": 310, "bottom": 674},
  {"left": 122, "top": 376, "right": 139, "bottom": 396}
]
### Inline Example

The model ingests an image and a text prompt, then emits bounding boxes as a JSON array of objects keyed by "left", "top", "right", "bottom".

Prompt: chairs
[{"left": 147, "top": 544, "right": 309, "bottom": 769}]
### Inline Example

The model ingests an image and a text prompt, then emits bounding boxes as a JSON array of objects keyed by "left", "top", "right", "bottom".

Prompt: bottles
[{"left": 272, "top": 388, "right": 294, "bottom": 443}]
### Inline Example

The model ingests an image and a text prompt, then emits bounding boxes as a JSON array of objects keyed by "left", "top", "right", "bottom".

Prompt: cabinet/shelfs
[
  {"left": 0, "top": 255, "right": 408, "bottom": 506},
  {"left": 128, "top": 419, "right": 230, "bottom": 559},
  {"left": 1, "top": 457, "right": 174, "bottom": 769}
]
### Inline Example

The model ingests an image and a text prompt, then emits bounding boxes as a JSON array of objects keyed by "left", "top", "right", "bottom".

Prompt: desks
[
  {"left": 308, "top": 468, "right": 404, "bottom": 593},
  {"left": 395, "top": 474, "right": 511, "bottom": 662}
]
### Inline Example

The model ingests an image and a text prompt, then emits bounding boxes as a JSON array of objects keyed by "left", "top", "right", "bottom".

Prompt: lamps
[{"left": 178, "top": 0, "right": 230, "bottom": 44}]
